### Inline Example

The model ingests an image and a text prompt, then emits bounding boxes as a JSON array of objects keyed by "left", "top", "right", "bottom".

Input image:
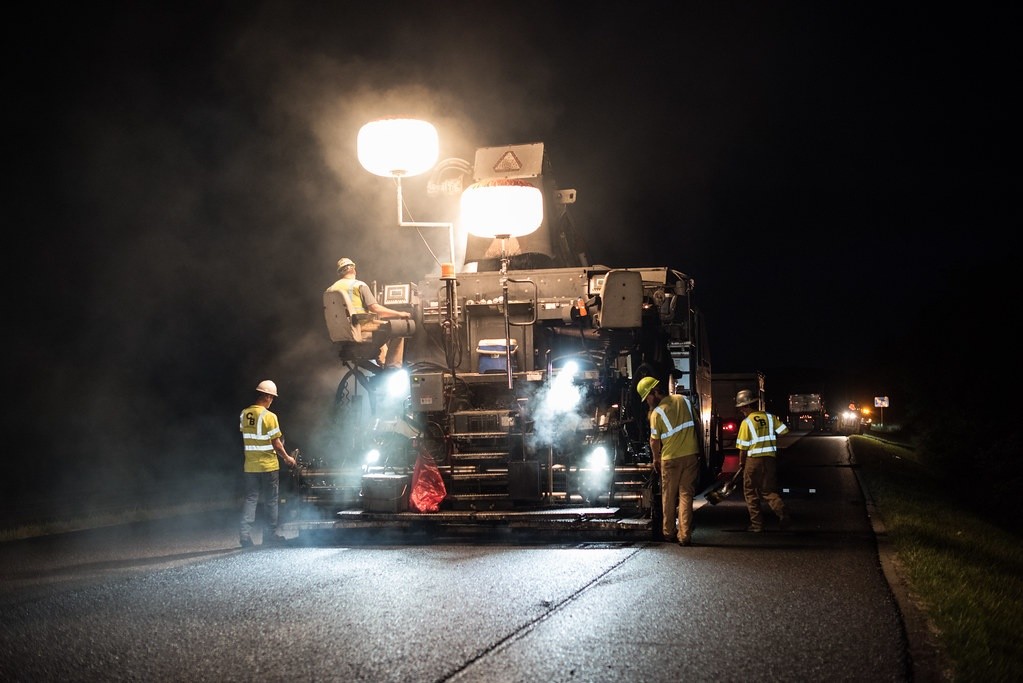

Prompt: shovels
[{"left": 704, "top": 466, "right": 743, "bottom": 506}]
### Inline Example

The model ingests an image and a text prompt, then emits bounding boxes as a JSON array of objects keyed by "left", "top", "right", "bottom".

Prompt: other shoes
[
  {"left": 678, "top": 541, "right": 689, "bottom": 546},
  {"left": 263, "top": 534, "right": 285, "bottom": 544},
  {"left": 241, "top": 538, "right": 254, "bottom": 547},
  {"left": 661, "top": 532, "right": 674, "bottom": 542},
  {"left": 746, "top": 525, "right": 762, "bottom": 532},
  {"left": 780, "top": 506, "right": 791, "bottom": 528}
]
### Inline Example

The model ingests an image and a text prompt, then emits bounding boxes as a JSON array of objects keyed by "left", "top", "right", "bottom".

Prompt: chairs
[
  {"left": 570, "top": 271, "right": 643, "bottom": 333},
  {"left": 322, "top": 291, "right": 373, "bottom": 343}
]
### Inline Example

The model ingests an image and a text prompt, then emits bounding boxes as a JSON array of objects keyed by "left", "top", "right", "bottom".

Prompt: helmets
[
  {"left": 637, "top": 376, "right": 660, "bottom": 403},
  {"left": 336, "top": 258, "right": 356, "bottom": 272},
  {"left": 256, "top": 380, "right": 278, "bottom": 397},
  {"left": 735, "top": 389, "right": 759, "bottom": 408}
]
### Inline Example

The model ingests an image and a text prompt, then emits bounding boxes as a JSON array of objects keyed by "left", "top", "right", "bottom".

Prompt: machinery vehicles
[{"left": 288, "top": 119, "right": 765, "bottom": 541}]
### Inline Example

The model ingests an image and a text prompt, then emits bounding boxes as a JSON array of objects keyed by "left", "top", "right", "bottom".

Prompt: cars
[{"left": 812, "top": 419, "right": 833, "bottom": 432}]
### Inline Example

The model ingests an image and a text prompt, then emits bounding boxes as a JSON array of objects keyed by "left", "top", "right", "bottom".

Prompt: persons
[
  {"left": 636, "top": 374, "right": 702, "bottom": 547},
  {"left": 734, "top": 388, "right": 794, "bottom": 536},
  {"left": 239, "top": 377, "right": 299, "bottom": 548},
  {"left": 327, "top": 257, "right": 411, "bottom": 386}
]
